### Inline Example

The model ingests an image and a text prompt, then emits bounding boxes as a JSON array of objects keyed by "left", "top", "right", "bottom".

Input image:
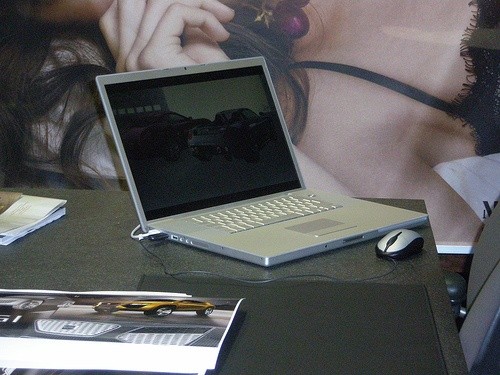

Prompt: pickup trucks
[{"left": 188, "top": 108, "right": 278, "bottom": 163}]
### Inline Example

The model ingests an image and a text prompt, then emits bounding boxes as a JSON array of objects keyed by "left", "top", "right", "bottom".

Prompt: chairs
[{"left": 459, "top": 196, "right": 500, "bottom": 370}]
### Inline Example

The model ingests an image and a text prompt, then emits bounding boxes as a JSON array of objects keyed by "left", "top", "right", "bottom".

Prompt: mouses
[{"left": 375, "top": 228, "right": 424, "bottom": 260}]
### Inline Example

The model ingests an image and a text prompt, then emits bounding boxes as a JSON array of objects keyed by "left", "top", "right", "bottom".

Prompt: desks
[{"left": 0, "top": 188, "right": 470, "bottom": 375}]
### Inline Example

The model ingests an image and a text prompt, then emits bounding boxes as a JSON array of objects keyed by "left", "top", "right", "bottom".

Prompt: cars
[
  {"left": 119, "top": 112, "right": 212, "bottom": 162},
  {"left": 94, "top": 298, "right": 214, "bottom": 318}
]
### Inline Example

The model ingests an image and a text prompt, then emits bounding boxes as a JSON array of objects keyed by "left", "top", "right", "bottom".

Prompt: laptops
[{"left": 95, "top": 56, "right": 428, "bottom": 267}]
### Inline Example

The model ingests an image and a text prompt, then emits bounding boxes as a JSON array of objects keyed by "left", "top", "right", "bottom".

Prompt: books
[{"left": 0, "top": 192, "right": 67, "bottom": 246}]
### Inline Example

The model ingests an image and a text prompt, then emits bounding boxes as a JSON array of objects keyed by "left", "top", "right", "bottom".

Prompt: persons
[{"left": 0, "top": 0, "right": 500, "bottom": 247}]
